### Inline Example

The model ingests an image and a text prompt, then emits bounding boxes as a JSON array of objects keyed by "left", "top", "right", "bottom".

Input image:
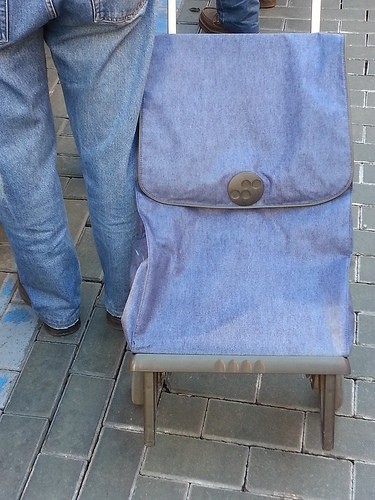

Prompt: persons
[
  {"left": 0, "top": 0, "right": 155, "bottom": 338},
  {"left": 195, "top": 0, "right": 279, "bottom": 37}
]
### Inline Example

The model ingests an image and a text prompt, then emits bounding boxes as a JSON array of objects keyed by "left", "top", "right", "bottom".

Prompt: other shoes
[{"left": 14, "top": 271, "right": 123, "bottom": 337}]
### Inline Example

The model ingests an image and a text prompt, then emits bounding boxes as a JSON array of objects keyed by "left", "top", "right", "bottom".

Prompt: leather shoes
[{"left": 199, "top": 0, "right": 277, "bottom": 33}]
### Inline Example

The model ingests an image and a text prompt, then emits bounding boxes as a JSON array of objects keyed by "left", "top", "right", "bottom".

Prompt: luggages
[{"left": 123, "top": 0, "right": 354, "bottom": 451}]
